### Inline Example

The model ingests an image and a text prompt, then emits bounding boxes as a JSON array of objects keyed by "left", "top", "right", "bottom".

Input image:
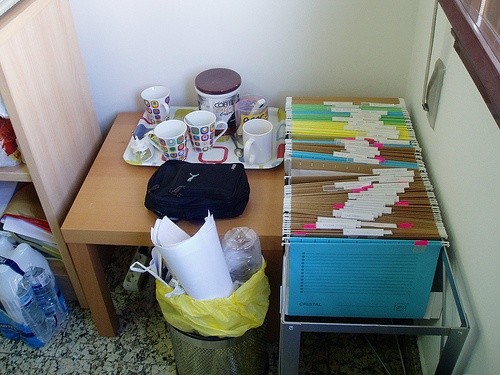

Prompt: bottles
[
  {"left": 195, "top": 68, "right": 244, "bottom": 137},
  {"left": 221, "top": 226, "right": 264, "bottom": 289},
  {"left": 0, "top": 230, "right": 69, "bottom": 350}
]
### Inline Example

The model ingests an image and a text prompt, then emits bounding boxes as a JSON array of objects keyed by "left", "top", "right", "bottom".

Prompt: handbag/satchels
[{"left": 145, "top": 159, "right": 251, "bottom": 222}]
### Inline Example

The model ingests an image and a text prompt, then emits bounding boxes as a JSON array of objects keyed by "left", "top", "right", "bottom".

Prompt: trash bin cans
[{"left": 150, "top": 254, "right": 278, "bottom": 375}]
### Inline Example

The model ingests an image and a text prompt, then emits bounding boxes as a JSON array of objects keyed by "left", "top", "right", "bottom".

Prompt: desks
[{"left": 61, "top": 112, "right": 285, "bottom": 337}]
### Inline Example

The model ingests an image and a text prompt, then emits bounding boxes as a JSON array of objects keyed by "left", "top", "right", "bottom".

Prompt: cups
[
  {"left": 183, "top": 110, "right": 228, "bottom": 152},
  {"left": 141, "top": 86, "right": 171, "bottom": 123},
  {"left": 146, "top": 120, "right": 188, "bottom": 161},
  {"left": 242, "top": 119, "right": 273, "bottom": 164}
]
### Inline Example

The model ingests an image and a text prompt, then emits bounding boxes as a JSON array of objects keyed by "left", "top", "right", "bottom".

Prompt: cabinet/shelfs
[{"left": 0, "top": 0, "right": 102, "bottom": 309}]
